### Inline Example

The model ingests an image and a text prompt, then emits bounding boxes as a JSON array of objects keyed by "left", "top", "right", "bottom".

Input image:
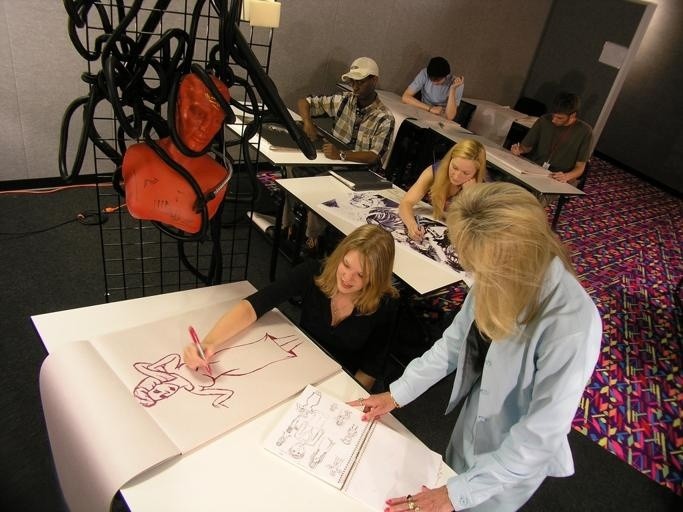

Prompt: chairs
[{"left": 377, "top": 110, "right": 417, "bottom": 173}]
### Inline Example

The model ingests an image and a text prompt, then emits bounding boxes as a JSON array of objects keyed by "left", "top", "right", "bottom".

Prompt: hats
[{"left": 341, "top": 57, "right": 379, "bottom": 82}]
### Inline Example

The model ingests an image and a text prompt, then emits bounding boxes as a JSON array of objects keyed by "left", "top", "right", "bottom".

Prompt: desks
[
  {"left": 335, "top": 83, "right": 591, "bottom": 231},
  {"left": 29, "top": 279, "right": 465, "bottom": 512},
  {"left": 269, "top": 171, "right": 466, "bottom": 294},
  {"left": 224, "top": 101, "right": 369, "bottom": 164}
]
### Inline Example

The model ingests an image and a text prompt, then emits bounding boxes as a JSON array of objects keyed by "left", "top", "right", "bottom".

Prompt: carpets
[{"left": 254, "top": 152, "right": 683, "bottom": 496}]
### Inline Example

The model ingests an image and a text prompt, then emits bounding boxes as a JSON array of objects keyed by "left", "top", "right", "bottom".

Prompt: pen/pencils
[
  {"left": 518, "top": 142, "right": 520, "bottom": 157},
  {"left": 189, "top": 325, "right": 212, "bottom": 375},
  {"left": 416, "top": 214, "right": 423, "bottom": 242}
]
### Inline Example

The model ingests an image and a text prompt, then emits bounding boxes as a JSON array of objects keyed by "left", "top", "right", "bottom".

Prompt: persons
[
  {"left": 297, "top": 56, "right": 395, "bottom": 249},
  {"left": 402, "top": 56, "right": 464, "bottom": 120},
  {"left": 344, "top": 180, "right": 602, "bottom": 512},
  {"left": 511, "top": 92, "right": 594, "bottom": 208},
  {"left": 398, "top": 139, "right": 487, "bottom": 243},
  {"left": 183, "top": 223, "right": 400, "bottom": 393},
  {"left": 121, "top": 73, "right": 228, "bottom": 232}
]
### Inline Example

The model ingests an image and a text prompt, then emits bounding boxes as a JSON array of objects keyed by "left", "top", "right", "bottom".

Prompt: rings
[
  {"left": 407, "top": 494, "right": 413, "bottom": 502},
  {"left": 408, "top": 501, "right": 416, "bottom": 510},
  {"left": 414, "top": 505, "right": 423, "bottom": 512},
  {"left": 194, "top": 367, "right": 199, "bottom": 372}
]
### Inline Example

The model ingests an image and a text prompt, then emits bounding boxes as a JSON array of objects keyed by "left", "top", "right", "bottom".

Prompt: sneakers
[
  {"left": 299, "top": 236, "right": 320, "bottom": 262},
  {"left": 266, "top": 226, "right": 306, "bottom": 240}
]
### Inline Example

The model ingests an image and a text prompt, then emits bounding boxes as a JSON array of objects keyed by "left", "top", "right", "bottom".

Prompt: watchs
[{"left": 340, "top": 150, "right": 346, "bottom": 160}]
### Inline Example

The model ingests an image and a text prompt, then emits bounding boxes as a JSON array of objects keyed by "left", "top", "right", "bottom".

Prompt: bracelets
[{"left": 428, "top": 105, "right": 434, "bottom": 112}]
